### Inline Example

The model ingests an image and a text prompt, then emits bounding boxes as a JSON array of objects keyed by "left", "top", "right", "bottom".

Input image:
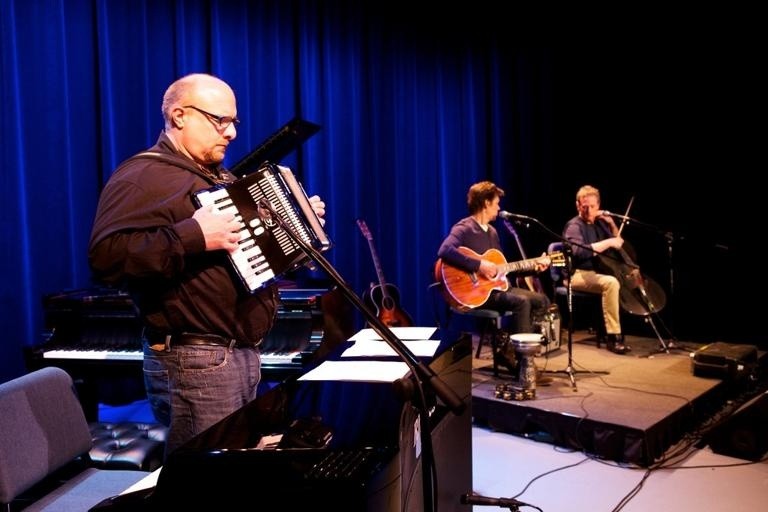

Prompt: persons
[
  {"left": 437, "top": 180, "right": 554, "bottom": 386},
  {"left": 88, "top": 72, "right": 326, "bottom": 456},
  {"left": 562, "top": 184, "right": 632, "bottom": 354}
]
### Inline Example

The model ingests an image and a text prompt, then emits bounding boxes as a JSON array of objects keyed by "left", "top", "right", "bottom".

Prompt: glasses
[{"left": 183, "top": 104, "right": 241, "bottom": 129}]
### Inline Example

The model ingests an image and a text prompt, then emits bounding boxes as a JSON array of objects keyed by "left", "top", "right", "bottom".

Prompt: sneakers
[{"left": 498, "top": 343, "right": 554, "bottom": 386}]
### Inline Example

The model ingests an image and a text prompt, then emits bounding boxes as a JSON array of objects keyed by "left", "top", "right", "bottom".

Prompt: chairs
[
  {"left": 441, "top": 304, "right": 516, "bottom": 379},
  {"left": 1, "top": 365, "right": 155, "bottom": 512},
  {"left": 547, "top": 241, "right": 604, "bottom": 349}
]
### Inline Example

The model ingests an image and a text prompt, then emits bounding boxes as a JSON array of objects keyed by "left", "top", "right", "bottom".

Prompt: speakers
[{"left": 696, "top": 383, "right": 768, "bottom": 462}]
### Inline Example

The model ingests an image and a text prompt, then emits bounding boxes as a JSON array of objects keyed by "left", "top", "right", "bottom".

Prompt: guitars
[
  {"left": 435, "top": 247, "right": 552, "bottom": 313},
  {"left": 356, "top": 219, "right": 413, "bottom": 327}
]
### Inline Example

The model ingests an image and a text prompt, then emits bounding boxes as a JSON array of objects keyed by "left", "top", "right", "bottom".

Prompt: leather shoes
[{"left": 607, "top": 335, "right": 632, "bottom": 353}]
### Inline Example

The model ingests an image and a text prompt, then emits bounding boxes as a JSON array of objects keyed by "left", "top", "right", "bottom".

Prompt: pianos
[{"left": 36, "top": 275, "right": 356, "bottom": 422}]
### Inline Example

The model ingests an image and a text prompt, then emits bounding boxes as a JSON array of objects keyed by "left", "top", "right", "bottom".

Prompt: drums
[{"left": 510, "top": 333, "right": 545, "bottom": 392}]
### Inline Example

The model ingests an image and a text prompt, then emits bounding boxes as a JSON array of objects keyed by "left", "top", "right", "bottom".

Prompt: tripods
[
  {"left": 638, "top": 233, "right": 700, "bottom": 359},
  {"left": 538, "top": 244, "right": 610, "bottom": 393}
]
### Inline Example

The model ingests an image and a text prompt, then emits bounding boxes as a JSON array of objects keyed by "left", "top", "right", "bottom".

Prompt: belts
[{"left": 144, "top": 327, "right": 248, "bottom": 350}]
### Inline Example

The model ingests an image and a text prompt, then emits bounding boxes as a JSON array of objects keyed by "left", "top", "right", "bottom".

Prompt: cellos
[{"left": 592, "top": 211, "right": 665, "bottom": 322}]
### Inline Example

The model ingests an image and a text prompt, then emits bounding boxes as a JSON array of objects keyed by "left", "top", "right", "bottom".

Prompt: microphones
[
  {"left": 460, "top": 494, "right": 524, "bottom": 508},
  {"left": 603, "top": 209, "right": 631, "bottom": 222}
]
